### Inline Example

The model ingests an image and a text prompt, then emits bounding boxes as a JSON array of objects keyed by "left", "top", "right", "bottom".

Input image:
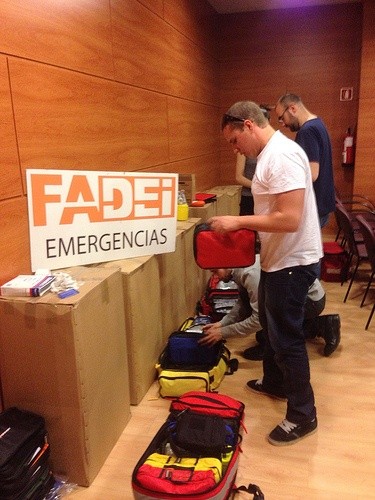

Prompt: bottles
[
  {"left": 178, "top": 191, "right": 183, "bottom": 205},
  {"left": 180, "top": 189, "right": 187, "bottom": 205}
]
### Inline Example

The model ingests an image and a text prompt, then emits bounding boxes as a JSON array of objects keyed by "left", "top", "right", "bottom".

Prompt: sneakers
[
  {"left": 267, "top": 415, "right": 318, "bottom": 447},
  {"left": 247, "top": 377, "right": 288, "bottom": 402}
]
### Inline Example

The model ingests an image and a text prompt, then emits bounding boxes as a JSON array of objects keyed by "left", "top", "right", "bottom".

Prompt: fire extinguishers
[{"left": 342, "top": 127, "right": 354, "bottom": 166}]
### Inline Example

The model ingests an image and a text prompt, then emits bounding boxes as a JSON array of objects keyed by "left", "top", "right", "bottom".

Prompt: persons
[
  {"left": 276, "top": 94, "right": 335, "bottom": 227},
  {"left": 235, "top": 105, "right": 272, "bottom": 215},
  {"left": 200, "top": 255, "right": 341, "bottom": 360},
  {"left": 206, "top": 102, "right": 324, "bottom": 446}
]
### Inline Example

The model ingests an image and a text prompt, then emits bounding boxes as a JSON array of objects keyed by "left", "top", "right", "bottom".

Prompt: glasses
[
  {"left": 222, "top": 113, "right": 245, "bottom": 130},
  {"left": 278, "top": 103, "right": 291, "bottom": 122}
]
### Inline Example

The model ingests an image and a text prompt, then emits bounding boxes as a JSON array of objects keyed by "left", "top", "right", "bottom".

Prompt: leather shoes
[
  {"left": 243, "top": 345, "right": 264, "bottom": 361},
  {"left": 323, "top": 314, "right": 341, "bottom": 357}
]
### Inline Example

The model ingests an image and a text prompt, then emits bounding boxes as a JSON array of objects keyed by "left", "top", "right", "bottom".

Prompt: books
[
  {"left": 1, "top": 274, "right": 57, "bottom": 297},
  {"left": 195, "top": 193, "right": 217, "bottom": 203}
]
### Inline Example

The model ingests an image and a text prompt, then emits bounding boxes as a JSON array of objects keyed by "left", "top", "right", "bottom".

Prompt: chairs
[{"left": 334, "top": 189, "right": 375, "bottom": 330}]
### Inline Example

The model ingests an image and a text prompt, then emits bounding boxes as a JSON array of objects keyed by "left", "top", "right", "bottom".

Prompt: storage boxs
[{"left": 1, "top": 185, "right": 242, "bottom": 487}]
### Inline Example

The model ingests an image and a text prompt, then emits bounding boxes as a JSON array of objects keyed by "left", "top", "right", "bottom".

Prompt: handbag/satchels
[
  {"left": 1, "top": 406, "right": 54, "bottom": 500},
  {"left": 155, "top": 317, "right": 238, "bottom": 399},
  {"left": 319, "top": 242, "right": 349, "bottom": 282},
  {"left": 193, "top": 223, "right": 261, "bottom": 269},
  {"left": 131, "top": 391, "right": 267, "bottom": 500}
]
